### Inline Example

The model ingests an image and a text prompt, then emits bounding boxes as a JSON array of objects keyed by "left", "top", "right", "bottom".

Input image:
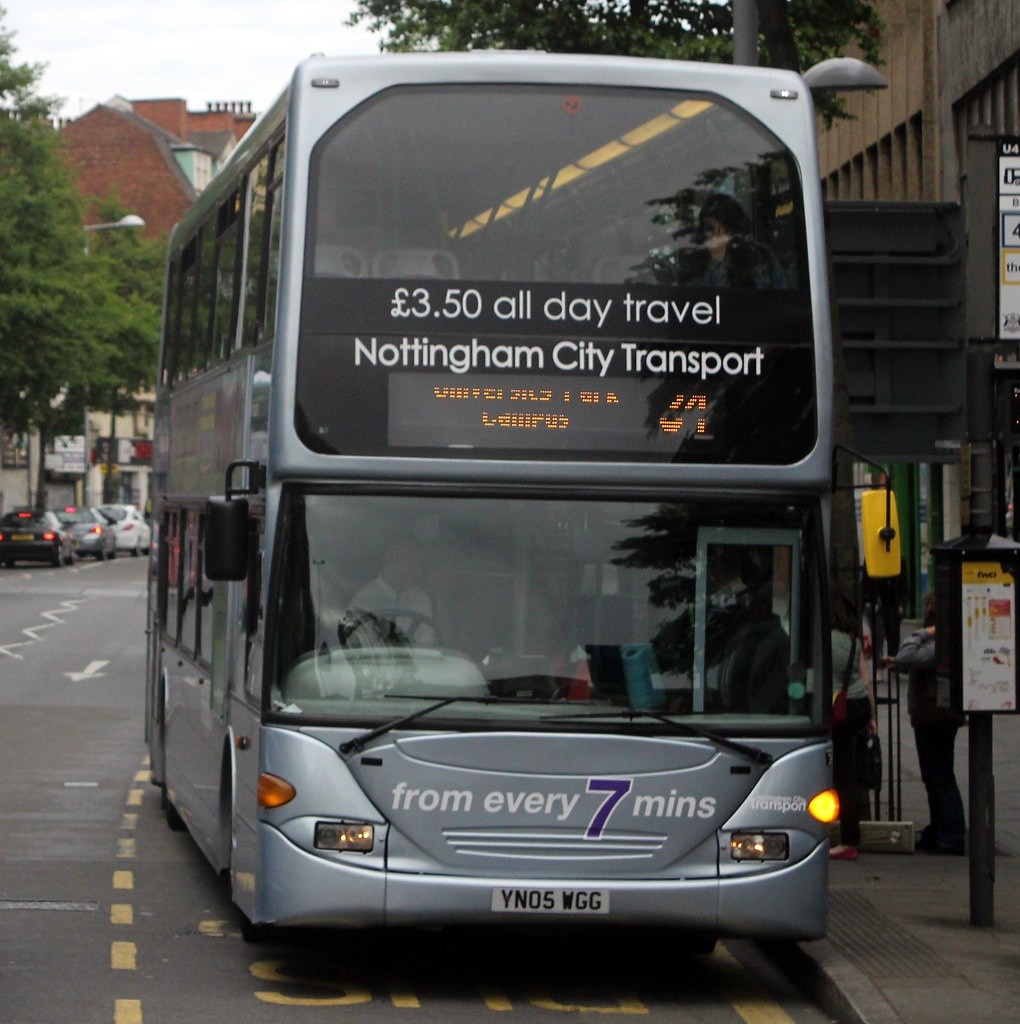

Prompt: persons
[
  {"left": 896, "top": 599, "right": 965, "bottom": 841},
  {"left": 529, "top": 597, "right": 593, "bottom": 689},
  {"left": 634, "top": 214, "right": 732, "bottom": 286},
  {"left": 344, "top": 535, "right": 435, "bottom": 645},
  {"left": 829, "top": 596, "right": 877, "bottom": 859}
]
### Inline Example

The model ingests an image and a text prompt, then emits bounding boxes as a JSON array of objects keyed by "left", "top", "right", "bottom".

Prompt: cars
[{"left": 0, "top": 504, "right": 151, "bottom": 567}]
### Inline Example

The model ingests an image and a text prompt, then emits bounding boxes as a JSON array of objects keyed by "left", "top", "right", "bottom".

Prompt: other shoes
[{"left": 829, "top": 846, "right": 859, "bottom": 860}]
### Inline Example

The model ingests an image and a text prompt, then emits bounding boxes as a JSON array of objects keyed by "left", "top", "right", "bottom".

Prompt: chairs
[{"left": 316, "top": 245, "right": 642, "bottom": 280}]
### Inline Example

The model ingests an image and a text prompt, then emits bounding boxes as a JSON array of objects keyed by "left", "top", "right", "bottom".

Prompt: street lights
[{"left": 82, "top": 213, "right": 145, "bottom": 435}]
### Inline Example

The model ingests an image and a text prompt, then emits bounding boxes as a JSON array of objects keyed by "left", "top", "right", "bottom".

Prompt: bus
[{"left": 144, "top": 52, "right": 901, "bottom": 949}]
